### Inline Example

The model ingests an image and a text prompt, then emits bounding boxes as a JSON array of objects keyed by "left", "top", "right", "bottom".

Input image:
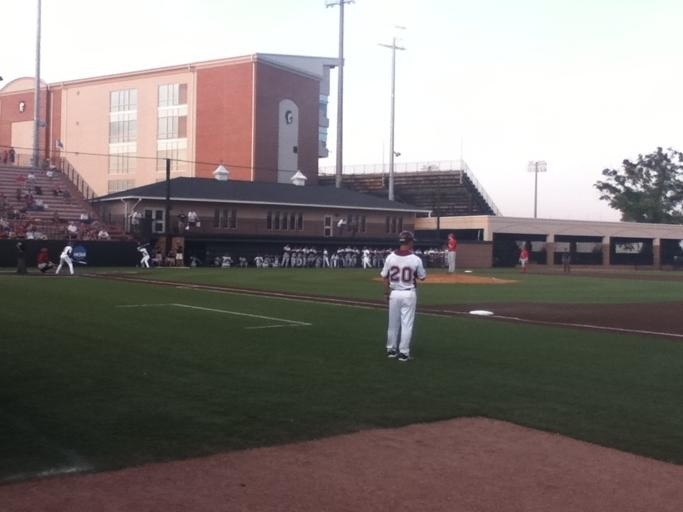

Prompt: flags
[{"left": 54, "top": 138, "right": 63, "bottom": 149}]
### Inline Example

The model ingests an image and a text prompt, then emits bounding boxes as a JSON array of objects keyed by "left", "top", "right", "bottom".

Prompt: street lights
[
  {"left": 379, "top": 38, "right": 408, "bottom": 200},
  {"left": 325, "top": 0, "right": 356, "bottom": 189},
  {"left": 527, "top": 160, "right": 548, "bottom": 219}
]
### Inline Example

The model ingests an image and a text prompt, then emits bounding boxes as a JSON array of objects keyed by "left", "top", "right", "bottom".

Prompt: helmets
[{"left": 399, "top": 231, "right": 417, "bottom": 243}]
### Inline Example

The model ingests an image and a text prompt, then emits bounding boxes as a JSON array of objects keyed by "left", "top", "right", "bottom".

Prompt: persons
[
  {"left": 164, "top": 248, "right": 176, "bottom": 266},
  {"left": 36, "top": 246, "right": 54, "bottom": 273},
  {"left": 16, "top": 236, "right": 28, "bottom": 275},
  {"left": 186, "top": 207, "right": 199, "bottom": 232},
  {"left": 281, "top": 244, "right": 448, "bottom": 272},
  {"left": 175, "top": 241, "right": 183, "bottom": 265},
  {"left": 136, "top": 244, "right": 152, "bottom": 270},
  {"left": 518, "top": 245, "right": 529, "bottom": 273},
  {"left": 55, "top": 241, "right": 74, "bottom": 275},
  {"left": 380, "top": 230, "right": 427, "bottom": 361},
  {"left": 561, "top": 248, "right": 571, "bottom": 272},
  {"left": 446, "top": 234, "right": 457, "bottom": 274},
  {"left": 150, "top": 246, "right": 162, "bottom": 265},
  {"left": 0, "top": 145, "right": 111, "bottom": 242},
  {"left": 212, "top": 254, "right": 282, "bottom": 269}
]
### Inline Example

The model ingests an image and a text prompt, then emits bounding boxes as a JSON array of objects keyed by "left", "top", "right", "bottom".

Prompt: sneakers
[
  {"left": 398, "top": 353, "right": 409, "bottom": 361},
  {"left": 388, "top": 349, "right": 399, "bottom": 358}
]
